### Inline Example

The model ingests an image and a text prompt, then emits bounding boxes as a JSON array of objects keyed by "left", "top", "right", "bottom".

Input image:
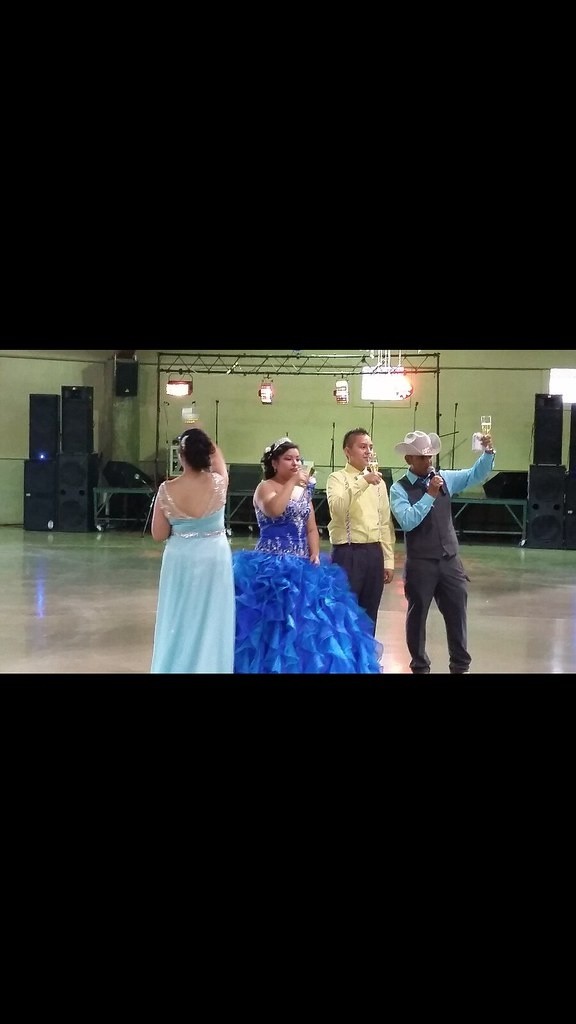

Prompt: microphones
[
  {"left": 427, "top": 466, "right": 447, "bottom": 497},
  {"left": 134, "top": 474, "right": 147, "bottom": 484}
]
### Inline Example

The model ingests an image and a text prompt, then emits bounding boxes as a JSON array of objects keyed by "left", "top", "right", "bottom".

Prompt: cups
[
  {"left": 367, "top": 454, "right": 379, "bottom": 474},
  {"left": 295, "top": 468, "right": 308, "bottom": 486}
]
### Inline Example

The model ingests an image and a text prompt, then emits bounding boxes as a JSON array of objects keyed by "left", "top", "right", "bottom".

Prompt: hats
[{"left": 395, "top": 431, "right": 441, "bottom": 455}]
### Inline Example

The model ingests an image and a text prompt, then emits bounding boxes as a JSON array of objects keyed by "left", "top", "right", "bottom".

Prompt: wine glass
[{"left": 480, "top": 416, "right": 493, "bottom": 451}]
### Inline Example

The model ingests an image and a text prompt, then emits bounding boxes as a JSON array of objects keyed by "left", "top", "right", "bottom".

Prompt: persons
[
  {"left": 232, "top": 438, "right": 386, "bottom": 673},
  {"left": 325, "top": 428, "right": 397, "bottom": 638},
  {"left": 151, "top": 428, "right": 236, "bottom": 673},
  {"left": 389, "top": 430, "right": 497, "bottom": 674}
]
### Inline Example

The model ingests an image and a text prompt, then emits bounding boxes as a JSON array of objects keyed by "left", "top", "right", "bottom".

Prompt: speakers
[
  {"left": 23, "top": 359, "right": 155, "bottom": 534},
  {"left": 368, "top": 469, "right": 393, "bottom": 496},
  {"left": 481, "top": 394, "right": 576, "bottom": 550},
  {"left": 229, "top": 464, "right": 265, "bottom": 493}
]
